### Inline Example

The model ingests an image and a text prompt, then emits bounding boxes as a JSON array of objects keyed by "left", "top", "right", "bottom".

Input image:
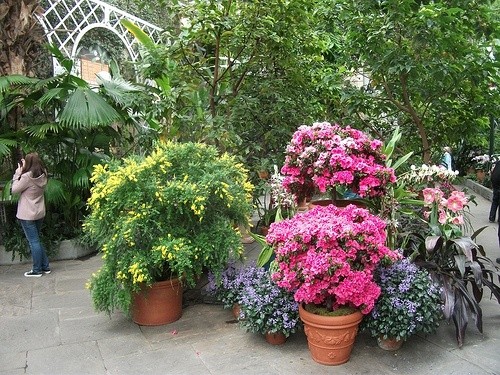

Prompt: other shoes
[{"left": 496, "top": 258, "right": 500, "bottom": 264}]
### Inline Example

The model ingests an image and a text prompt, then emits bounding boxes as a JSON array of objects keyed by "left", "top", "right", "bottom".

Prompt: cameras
[{"left": 17, "top": 160, "right": 23, "bottom": 167}]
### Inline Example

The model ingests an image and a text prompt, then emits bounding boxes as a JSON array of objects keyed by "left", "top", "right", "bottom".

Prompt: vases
[
  {"left": 263, "top": 326, "right": 288, "bottom": 345},
  {"left": 376, "top": 331, "right": 404, "bottom": 351},
  {"left": 299, "top": 299, "right": 365, "bottom": 366}
]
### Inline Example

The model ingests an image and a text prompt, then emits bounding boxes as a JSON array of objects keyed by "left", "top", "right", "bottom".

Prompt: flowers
[{"left": 208, "top": 123, "right": 500, "bottom": 347}]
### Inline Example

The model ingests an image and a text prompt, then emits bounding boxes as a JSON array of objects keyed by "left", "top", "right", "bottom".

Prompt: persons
[
  {"left": 12, "top": 152, "right": 51, "bottom": 277},
  {"left": 489, "top": 159, "right": 500, "bottom": 264},
  {"left": 440, "top": 147, "right": 451, "bottom": 171}
]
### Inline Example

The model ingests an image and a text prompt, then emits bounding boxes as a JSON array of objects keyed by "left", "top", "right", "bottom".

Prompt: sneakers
[
  {"left": 43, "top": 270, "right": 51, "bottom": 274},
  {"left": 24, "top": 270, "right": 42, "bottom": 277}
]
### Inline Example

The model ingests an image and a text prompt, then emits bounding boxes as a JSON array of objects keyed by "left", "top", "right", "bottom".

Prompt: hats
[{"left": 444, "top": 146, "right": 449, "bottom": 152}]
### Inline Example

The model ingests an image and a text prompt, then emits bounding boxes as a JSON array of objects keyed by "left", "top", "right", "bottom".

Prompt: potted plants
[{"left": 80, "top": 138, "right": 256, "bottom": 325}]
